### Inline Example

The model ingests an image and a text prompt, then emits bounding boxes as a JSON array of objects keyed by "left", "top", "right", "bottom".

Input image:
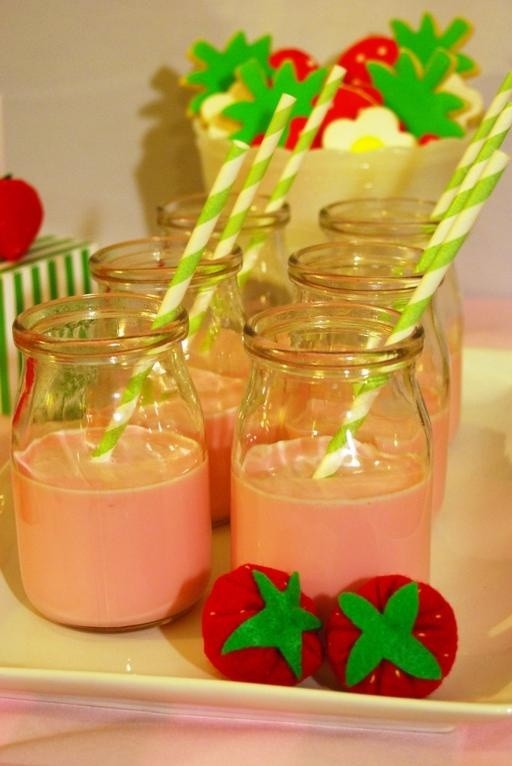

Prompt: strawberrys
[
  {"left": 250, "top": 36, "right": 439, "bottom": 152},
  {"left": 0, "top": 169, "right": 46, "bottom": 265},
  {"left": 202, "top": 560, "right": 326, "bottom": 687},
  {"left": 330, "top": 574, "right": 461, "bottom": 701}
]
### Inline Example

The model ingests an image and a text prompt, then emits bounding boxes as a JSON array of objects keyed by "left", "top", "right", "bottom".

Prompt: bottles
[
  {"left": 321, "top": 195, "right": 462, "bottom": 433},
  {"left": 89, "top": 235, "right": 246, "bottom": 523},
  {"left": 8, "top": 294, "right": 213, "bottom": 631},
  {"left": 160, "top": 188, "right": 292, "bottom": 328},
  {"left": 289, "top": 236, "right": 448, "bottom": 514},
  {"left": 232, "top": 305, "right": 430, "bottom": 594}
]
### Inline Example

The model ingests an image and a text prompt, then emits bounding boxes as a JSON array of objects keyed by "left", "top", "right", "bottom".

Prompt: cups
[{"left": 189, "top": 120, "right": 479, "bottom": 247}]
